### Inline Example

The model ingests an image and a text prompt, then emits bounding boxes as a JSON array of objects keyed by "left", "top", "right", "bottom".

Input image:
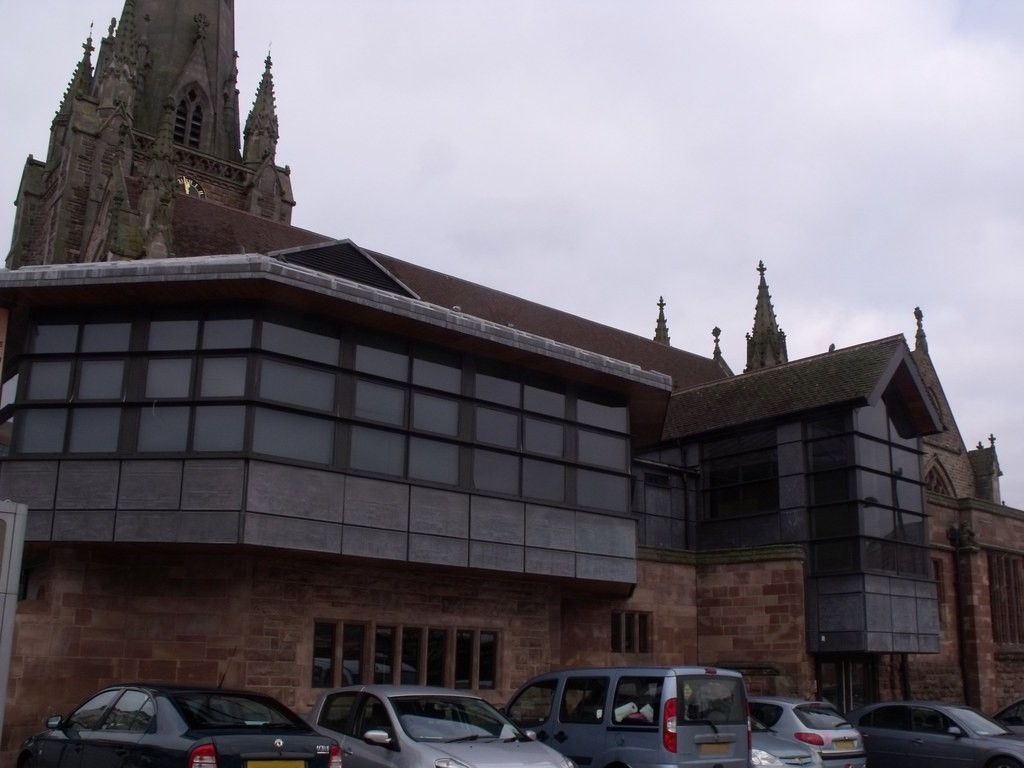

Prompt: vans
[{"left": 498, "top": 665, "right": 752, "bottom": 768}]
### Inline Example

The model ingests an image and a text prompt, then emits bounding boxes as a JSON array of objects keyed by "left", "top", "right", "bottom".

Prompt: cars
[
  {"left": 750, "top": 715, "right": 825, "bottom": 768},
  {"left": 747, "top": 697, "right": 867, "bottom": 768},
  {"left": 305, "top": 683, "right": 577, "bottom": 768},
  {"left": 989, "top": 698, "right": 1024, "bottom": 736},
  {"left": 16, "top": 683, "right": 343, "bottom": 768},
  {"left": 842, "top": 701, "right": 1024, "bottom": 768}
]
